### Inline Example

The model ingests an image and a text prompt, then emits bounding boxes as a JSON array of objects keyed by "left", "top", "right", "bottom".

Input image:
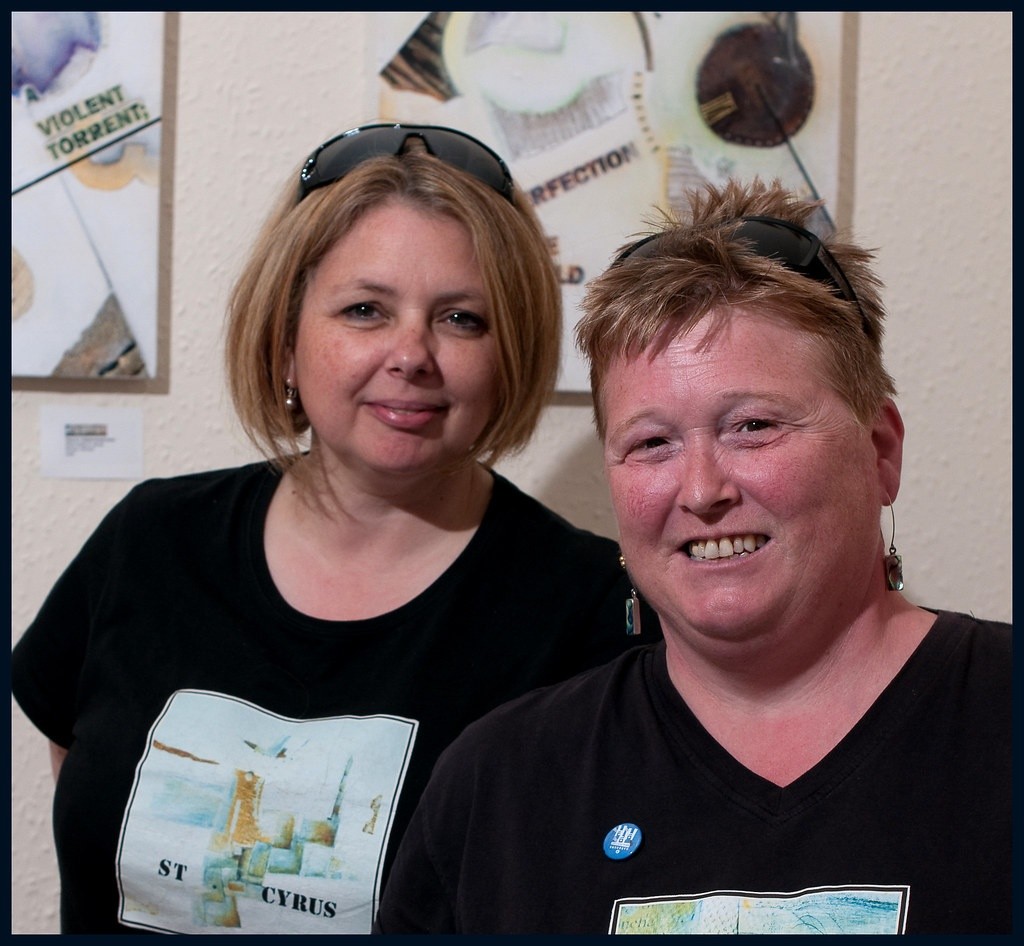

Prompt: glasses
[
  {"left": 605, "top": 215, "right": 871, "bottom": 333},
  {"left": 292, "top": 124, "right": 520, "bottom": 214}
]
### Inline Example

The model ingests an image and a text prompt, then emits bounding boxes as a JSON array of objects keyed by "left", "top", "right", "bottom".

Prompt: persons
[
  {"left": 368, "top": 173, "right": 1012, "bottom": 934},
  {"left": 11, "top": 124, "right": 664, "bottom": 934}
]
